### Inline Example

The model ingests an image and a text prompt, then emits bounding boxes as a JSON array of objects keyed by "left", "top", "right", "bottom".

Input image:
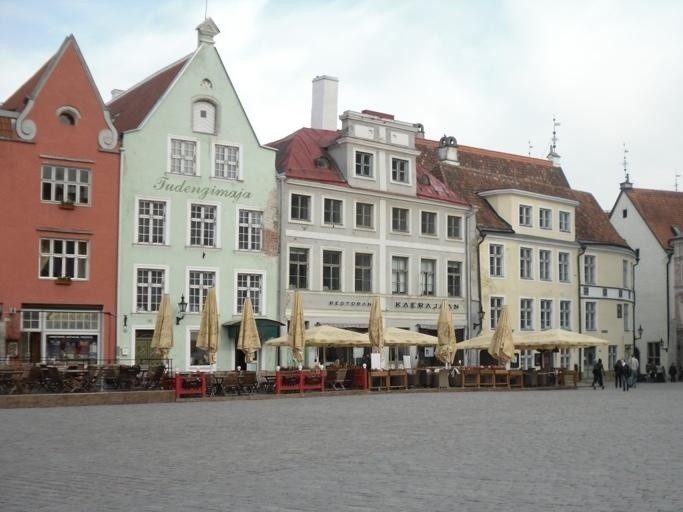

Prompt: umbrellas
[
  {"left": 266, "top": 324, "right": 364, "bottom": 370},
  {"left": 453, "top": 328, "right": 539, "bottom": 348},
  {"left": 363, "top": 326, "right": 436, "bottom": 366},
  {"left": 236, "top": 294, "right": 262, "bottom": 361},
  {"left": 150, "top": 292, "right": 175, "bottom": 360},
  {"left": 520, "top": 327, "right": 612, "bottom": 364},
  {"left": 195, "top": 286, "right": 218, "bottom": 374},
  {"left": 433, "top": 297, "right": 458, "bottom": 368},
  {"left": 488, "top": 305, "right": 513, "bottom": 367},
  {"left": 366, "top": 296, "right": 382, "bottom": 352},
  {"left": 286, "top": 289, "right": 305, "bottom": 365}
]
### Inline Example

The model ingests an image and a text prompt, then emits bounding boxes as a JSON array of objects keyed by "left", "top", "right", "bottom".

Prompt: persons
[
  {"left": 645, "top": 359, "right": 682, "bottom": 383},
  {"left": 628, "top": 353, "right": 639, "bottom": 387},
  {"left": 590, "top": 358, "right": 606, "bottom": 389},
  {"left": 612, "top": 359, "right": 622, "bottom": 388},
  {"left": 620, "top": 359, "right": 632, "bottom": 391}
]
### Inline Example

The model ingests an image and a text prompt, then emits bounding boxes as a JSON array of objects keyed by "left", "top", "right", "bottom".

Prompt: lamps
[
  {"left": 473, "top": 307, "right": 486, "bottom": 329},
  {"left": 176, "top": 293, "right": 188, "bottom": 325}
]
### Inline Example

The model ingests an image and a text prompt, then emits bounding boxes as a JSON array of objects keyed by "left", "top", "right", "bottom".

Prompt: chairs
[{"left": 0, "top": 360, "right": 578, "bottom": 402}]
